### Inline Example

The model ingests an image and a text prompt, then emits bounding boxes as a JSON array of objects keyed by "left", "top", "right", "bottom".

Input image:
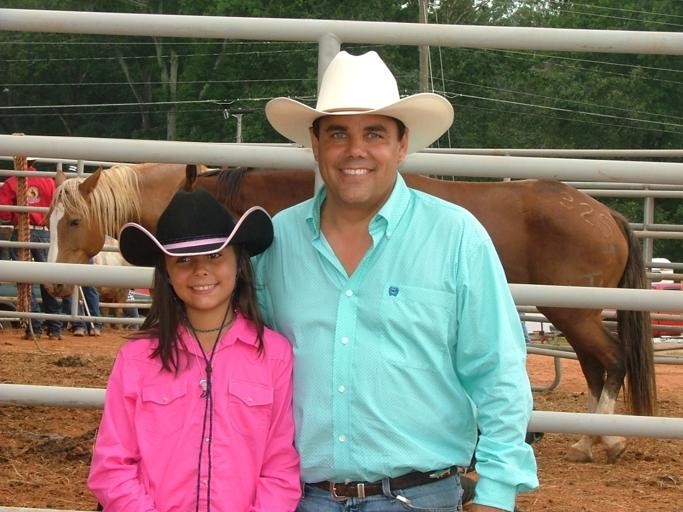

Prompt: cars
[
  {"left": 519, "top": 257, "right": 682, "bottom": 338},
  {"left": 0, "top": 223, "right": 159, "bottom": 331}
]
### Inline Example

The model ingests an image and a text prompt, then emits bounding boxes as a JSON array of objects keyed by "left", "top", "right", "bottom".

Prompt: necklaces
[
  {"left": 187, "top": 329, "right": 223, "bottom": 398},
  {"left": 188, "top": 316, "right": 234, "bottom": 333}
]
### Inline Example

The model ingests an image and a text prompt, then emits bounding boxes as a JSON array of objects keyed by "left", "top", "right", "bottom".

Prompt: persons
[
  {"left": 63, "top": 258, "right": 103, "bottom": 336},
  {"left": 0, "top": 161, "right": 63, "bottom": 339},
  {"left": 86, "top": 187, "right": 302, "bottom": 512},
  {"left": 250, "top": 49, "right": 540, "bottom": 512}
]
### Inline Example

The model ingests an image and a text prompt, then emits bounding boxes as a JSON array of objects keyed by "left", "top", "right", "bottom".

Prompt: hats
[
  {"left": 118, "top": 186, "right": 274, "bottom": 267},
  {"left": 264, "top": 50, "right": 454, "bottom": 156}
]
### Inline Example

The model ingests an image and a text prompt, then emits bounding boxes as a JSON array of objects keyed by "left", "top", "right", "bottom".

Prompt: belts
[
  {"left": 14, "top": 223, "right": 49, "bottom": 232},
  {"left": 305, "top": 465, "right": 458, "bottom": 502}
]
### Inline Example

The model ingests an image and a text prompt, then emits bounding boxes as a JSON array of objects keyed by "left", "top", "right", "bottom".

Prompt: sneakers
[{"left": 24, "top": 326, "right": 100, "bottom": 340}]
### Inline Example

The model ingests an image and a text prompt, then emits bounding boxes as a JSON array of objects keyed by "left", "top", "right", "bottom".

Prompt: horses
[
  {"left": 167, "top": 160, "right": 660, "bottom": 465},
  {"left": 41, "top": 161, "right": 205, "bottom": 297}
]
